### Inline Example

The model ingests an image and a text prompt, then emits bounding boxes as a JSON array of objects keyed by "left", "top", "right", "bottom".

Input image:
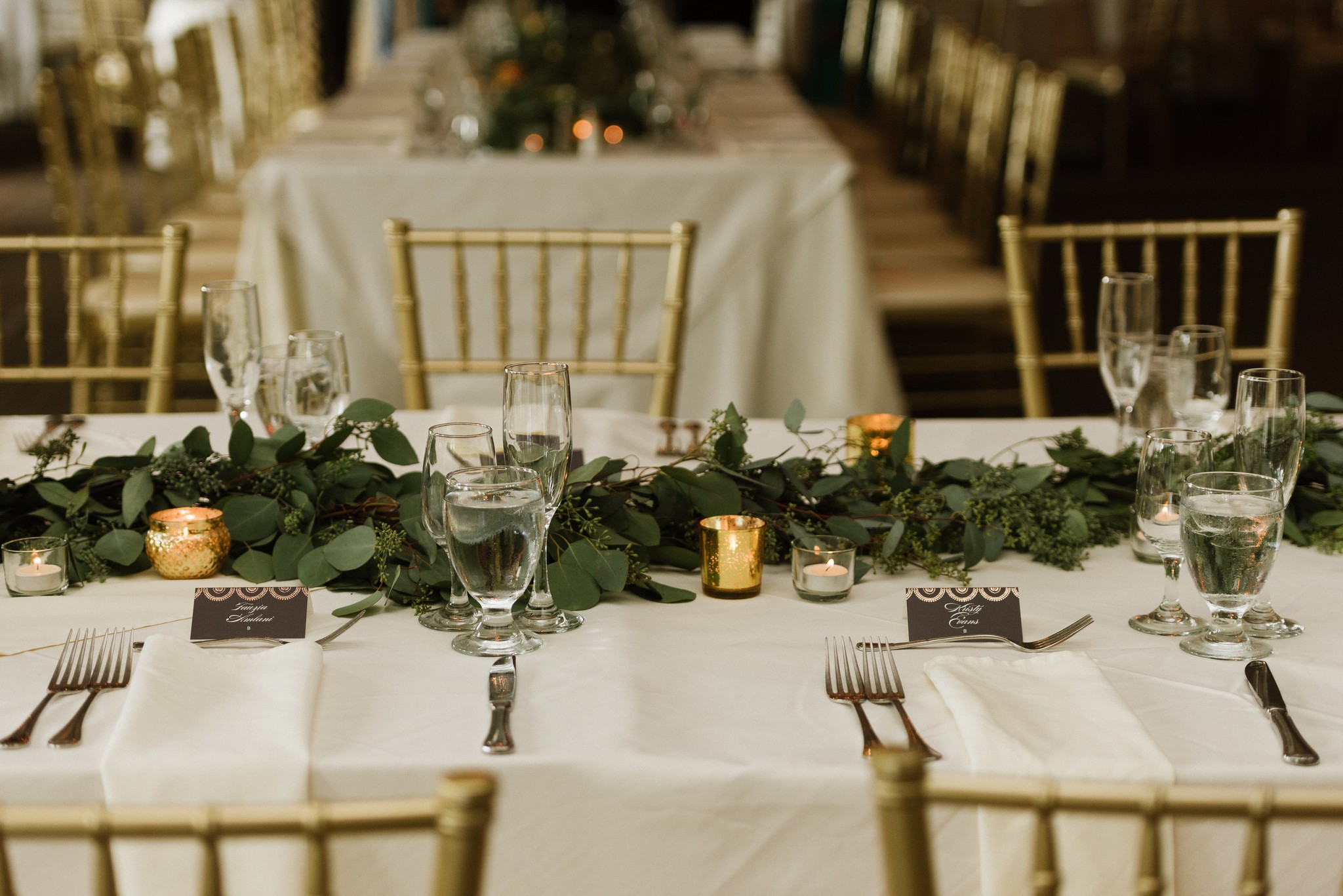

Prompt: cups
[
  {"left": 793, "top": 535, "right": 855, "bottom": 603},
  {"left": 1, "top": 536, "right": 69, "bottom": 597},
  {"left": 846, "top": 412, "right": 916, "bottom": 471},
  {"left": 701, "top": 515, "right": 765, "bottom": 599}
]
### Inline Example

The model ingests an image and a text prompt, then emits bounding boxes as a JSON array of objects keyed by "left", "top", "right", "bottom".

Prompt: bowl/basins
[{"left": 146, "top": 505, "right": 232, "bottom": 578}]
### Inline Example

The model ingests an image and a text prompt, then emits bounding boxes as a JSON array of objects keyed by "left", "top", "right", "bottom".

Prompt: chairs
[
  {"left": 0, "top": 767, "right": 502, "bottom": 895},
  {"left": 386, "top": 215, "right": 699, "bottom": 417},
  {"left": 28, "top": 1, "right": 328, "bottom": 413},
  {"left": 790, "top": 1, "right": 1066, "bottom": 419},
  {"left": 5, "top": 226, "right": 190, "bottom": 413},
  {"left": 997, "top": 206, "right": 1299, "bottom": 419},
  {"left": 870, "top": 745, "right": 1343, "bottom": 893}
]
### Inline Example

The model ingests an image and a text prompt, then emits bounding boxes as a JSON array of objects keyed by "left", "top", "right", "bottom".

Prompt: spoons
[
  {"left": 655, "top": 420, "right": 710, "bottom": 457},
  {"left": 131, "top": 611, "right": 364, "bottom": 652}
]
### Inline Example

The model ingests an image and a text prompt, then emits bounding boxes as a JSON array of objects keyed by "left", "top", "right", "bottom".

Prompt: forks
[
  {"left": 863, "top": 637, "right": 943, "bottom": 763},
  {"left": 0, "top": 627, "right": 98, "bottom": 748},
  {"left": 46, "top": 625, "right": 135, "bottom": 748},
  {"left": 856, "top": 614, "right": 1095, "bottom": 652},
  {"left": 12, "top": 415, "right": 86, "bottom": 452},
  {"left": 824, "top": 636, "right": 886, "bottom": 757}
]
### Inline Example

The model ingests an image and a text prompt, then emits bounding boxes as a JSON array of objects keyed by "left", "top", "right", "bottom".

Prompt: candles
[
  {"left": 805, "top": 558, "right": 848, "bottom": 592},
  {"left": 15, "top": 557, "right": 61, "bottom": 592},
  {"left": 1154, "top": 507, "right": 1180, "bottom": 526}
]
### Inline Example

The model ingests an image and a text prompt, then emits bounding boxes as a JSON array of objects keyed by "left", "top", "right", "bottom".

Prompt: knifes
[
  {"left": 481, "top": 655, "right": 518, "bottom": 756},
  {"left": 1244, "top": 661, "right": 1320, "bottom": 766}
]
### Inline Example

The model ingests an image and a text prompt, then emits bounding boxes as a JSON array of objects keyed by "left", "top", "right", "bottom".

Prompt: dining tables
[
  {"left": 239, "top": 19, "right": 900, "bottom": 419},
  {"left": 1, "top": 407, "right": 1343, "bottom": 896}
]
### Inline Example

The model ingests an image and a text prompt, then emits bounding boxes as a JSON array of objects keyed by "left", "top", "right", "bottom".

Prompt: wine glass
[
  {"left": 443, "top": 468, "right": 540, "bottom": 656},
  {"left": 284, "top": 328, "right": 352, "bottom": 438},
  {"left": 501, "top": 362, "right": 586, "bottom": 635},
  {"left": 202, "top": 279, "right": 262, "bottom": 426},
  {"left": 419, "top": 420, "right": 498, "bottom": 631},
  {"left": 1103, "top": 269, "right": 1303, "bottom": 660},
  {"left": 258, "top": 336, "right": 337, "bottom": 445}
]
